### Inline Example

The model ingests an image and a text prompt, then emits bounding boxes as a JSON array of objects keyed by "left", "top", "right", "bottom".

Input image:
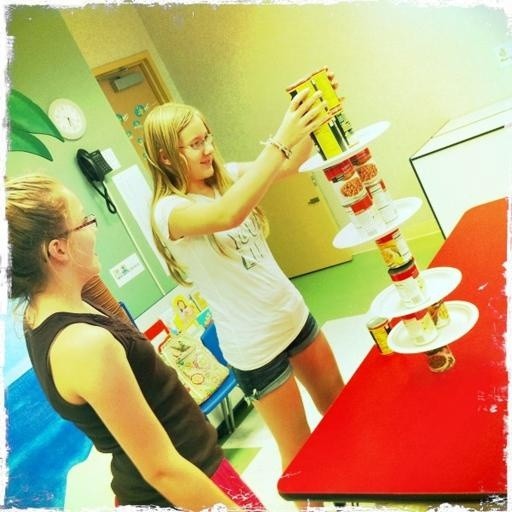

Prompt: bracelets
[{"left": 268, "top": 137, "right": 293, "bottom": 159}]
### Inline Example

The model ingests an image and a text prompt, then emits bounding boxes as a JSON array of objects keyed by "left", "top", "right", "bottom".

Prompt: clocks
[{"left": 48, "top": 99, "right": 86, "bottom": 141}]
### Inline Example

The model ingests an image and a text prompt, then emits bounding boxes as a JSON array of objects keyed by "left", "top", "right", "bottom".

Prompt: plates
[
  {"left": 385, "top": 299, "right": 480, "bottom": 356},
  {"left": 296, "top": 120, "right": 395, "bottom": 176},
  {"left": 368, "top": 265, "right": 464, "bottom": 320},
  {"left": 332, "top": 196, "right": 424, "bottom": 250}
]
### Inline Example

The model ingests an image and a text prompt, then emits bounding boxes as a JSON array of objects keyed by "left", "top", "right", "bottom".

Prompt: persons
[
  {"left": 177, "top": 299, "right": 195, "bottom": 321},
  {"left": 142, "top": 73, "right": 350, "bottom": 512},
  {"left": 6, "top": 175, "right": 268, "bottom": 512}
]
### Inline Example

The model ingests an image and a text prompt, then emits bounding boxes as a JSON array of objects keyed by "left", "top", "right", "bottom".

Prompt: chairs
[{"left": 198, "top": 321, "right": 237, "bottom": 434}]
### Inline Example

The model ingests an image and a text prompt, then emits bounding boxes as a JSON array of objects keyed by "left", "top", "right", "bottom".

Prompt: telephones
[{"left": 76, "top": 149, "right": 113, "bottom": 182}]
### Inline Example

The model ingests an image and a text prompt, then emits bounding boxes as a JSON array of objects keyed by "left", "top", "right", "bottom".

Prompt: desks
[
  {"left": 406, "top": 97, "right": 511, "bottom": 240},
  {"left": 276, "top": 198, "right": 511, "bottom": 510}
]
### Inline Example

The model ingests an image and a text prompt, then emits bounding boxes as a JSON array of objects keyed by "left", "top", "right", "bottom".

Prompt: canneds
[
  {"left": 376, "top": 228, "right": 413, "bottom": 271},
  {"left": 402, "top": 310, "right": 438, "bottom": 346},
  {"left": 388, "top": 258, "right": 430, "bottom": 309},
  {"left": 428, "top": 299, "right": 450, "bottom": 329},
  {"left": 285, "top": 66, "right": 360, "bottom": 162},
  {"left": 367, "top": 317, "right": 394, "bottom": 355},
  {"left": 424, "top": 345, "right": 456, "bottom": 373},
  {"left": 322, "top": 145, "right": 398, "bottom": 238}
]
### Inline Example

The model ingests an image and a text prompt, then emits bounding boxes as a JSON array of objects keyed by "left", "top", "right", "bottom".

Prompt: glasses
[
  {"left": 43, "top": 213, "right": 98, "bottom": 263},
  {"left": 179, "top": 133, "right": 214, "bottom": 150}
]
